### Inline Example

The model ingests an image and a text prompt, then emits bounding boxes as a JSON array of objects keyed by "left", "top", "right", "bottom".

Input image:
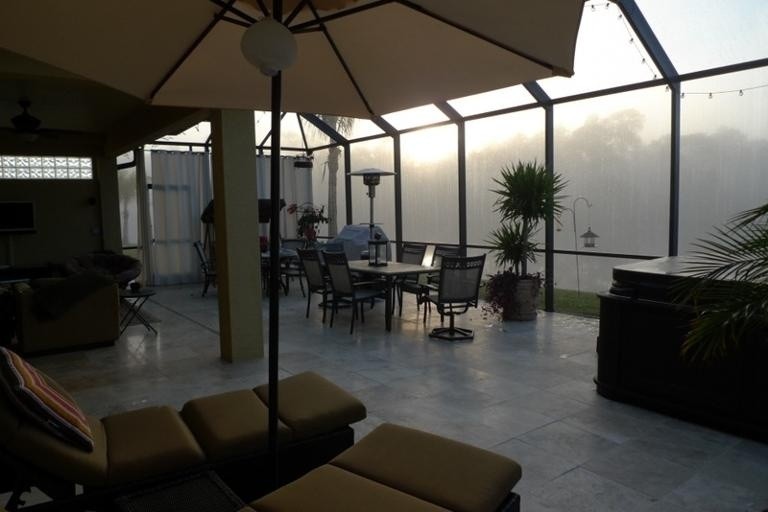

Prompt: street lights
[
  {"left": 349, "top": 166, "right": 396, "bottom": 250},
  {"left": 555, "top": 192, "right": 596, "bottom": 289}
]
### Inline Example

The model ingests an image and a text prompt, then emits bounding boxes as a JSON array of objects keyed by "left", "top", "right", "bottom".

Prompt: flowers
[{"left": 286, "top": 202, "right": 328, "bottom": 239}]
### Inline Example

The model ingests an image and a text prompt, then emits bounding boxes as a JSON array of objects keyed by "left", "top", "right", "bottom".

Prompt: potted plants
[{"left": 485, "top": 169, "right": 560, "bottom": 321}]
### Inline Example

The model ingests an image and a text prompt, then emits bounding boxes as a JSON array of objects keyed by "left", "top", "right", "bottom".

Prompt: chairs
[
  {"left": 62, "top": 254, "right": 142, "bottom": 289},
  {"left": 235, "top": 422, "right": 521, "bottom": 512},
  {"left": 0, "top": 346, "right": 366, "bottom": 512},
  {"left": 192, "top": 240, "right": 486, "bottom": 341},
  {"left": 0, "top": 278, "right": 120, "bottom": 358}
]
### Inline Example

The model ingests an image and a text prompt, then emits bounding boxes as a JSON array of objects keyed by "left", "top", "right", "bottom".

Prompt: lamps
[
  {"left": 239, "top": 18, "right": 298, "bottom": 76},
  {"left": 579, "top": 226, "right": 599, "bottom": 247}
]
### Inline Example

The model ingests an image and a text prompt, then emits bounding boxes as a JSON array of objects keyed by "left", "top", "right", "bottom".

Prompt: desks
[
  {"left": 119, "top": 288, "right": 157, "bottom": 335},
  {"left": 113, "top": 469, "right": 244, "bottom": 512}
]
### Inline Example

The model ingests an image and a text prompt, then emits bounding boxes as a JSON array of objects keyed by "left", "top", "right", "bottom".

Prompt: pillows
[{"left": 0, "top": 346, "right": 95, "bottom": 452}]
